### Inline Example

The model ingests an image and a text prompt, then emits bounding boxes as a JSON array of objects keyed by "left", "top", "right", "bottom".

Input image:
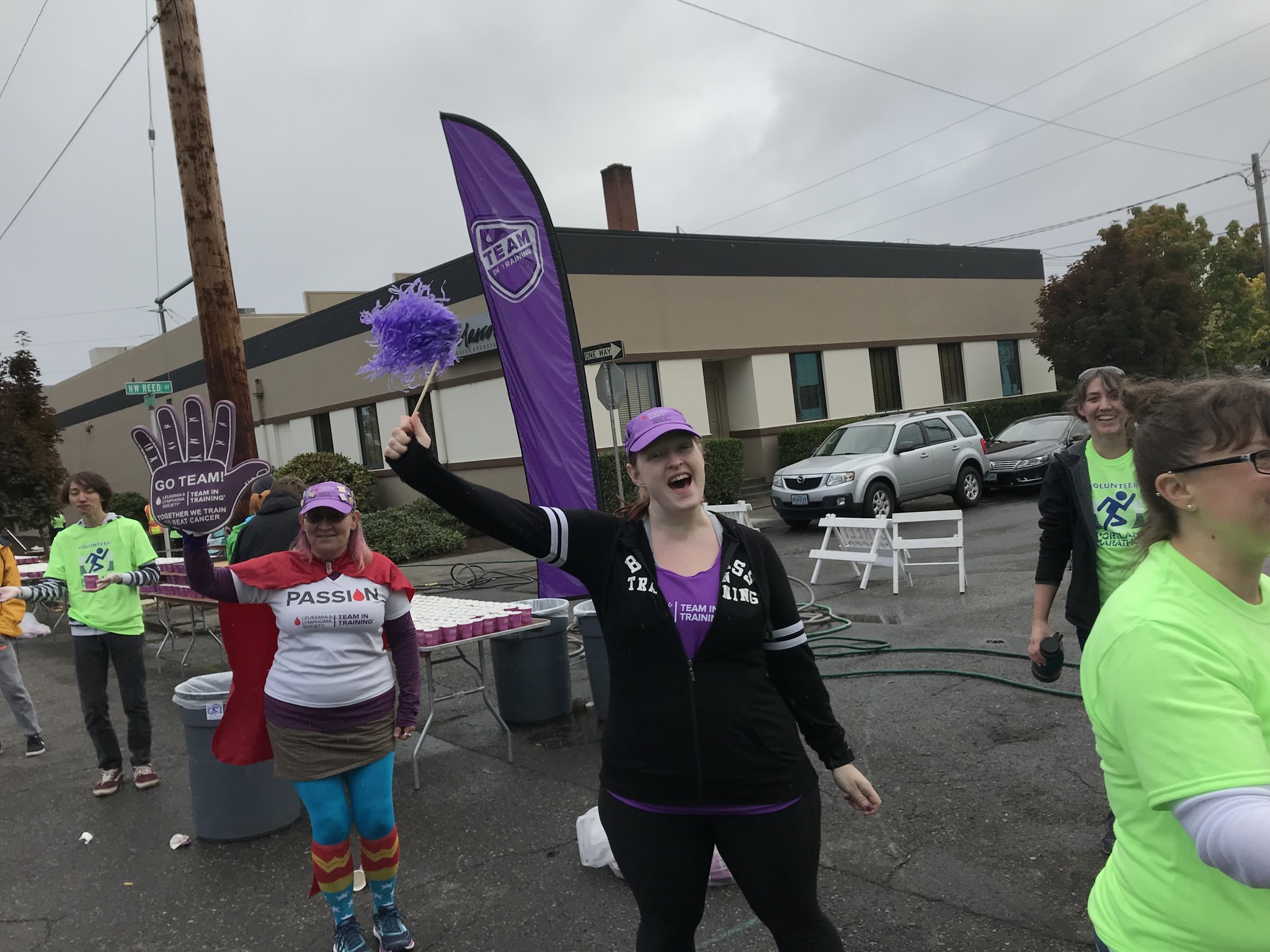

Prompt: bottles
[{"left": 1032, "top": 632, "right": 1064, "bottom": 683}]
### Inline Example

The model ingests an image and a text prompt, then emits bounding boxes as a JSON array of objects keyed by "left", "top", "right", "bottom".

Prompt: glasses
[
  {"left": 1170, "top": 449, "right": 1270, "bottom": 475},
  {"left": 1077, "top": 366, "right": 1124, "bottom": 383},
  {"left": 305, "top": 512, "right": 351, "bottom": 524}
]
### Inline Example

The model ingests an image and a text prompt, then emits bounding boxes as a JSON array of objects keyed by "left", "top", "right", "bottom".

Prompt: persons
[
  {"left": 177, "top": 475, "right": 419, "bottom": 952},
  {"left": 50, "top": 512, "right": 66, "bottom": 546},
  {"left": 0, "top": 471, "right": 161, "bottom": 798},
  {"left": 0, "top": 536, "right": 46, "bottom": 758},
  {"left": 386, "top": 406, "right": 883, "bottom": 952},
  {"left": 1027, "top": 366, "right": 1147, "bottom": 855},
  {"left": 1079, "top": 379, "right": 1270, "bottom": 952}
]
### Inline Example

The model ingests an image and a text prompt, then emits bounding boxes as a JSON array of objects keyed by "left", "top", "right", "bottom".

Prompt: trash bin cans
[
  {"left": 174, "top": 671, "right": 302, "bottom": 844},
  {"left": 573, "top": 599, "right": 610, "bottom": 721},
  {"left": 489, "top": 599, "right": 573, "bottom": 726}
]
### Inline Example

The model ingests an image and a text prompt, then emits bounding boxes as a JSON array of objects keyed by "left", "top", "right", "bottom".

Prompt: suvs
[{"left": 769, "top": 407, "right": 990, "bottom": 527}]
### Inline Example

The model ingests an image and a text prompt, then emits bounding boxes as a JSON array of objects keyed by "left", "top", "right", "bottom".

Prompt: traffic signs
[{"left": 581, "top": 340, "right": 625, "bottom": 366}]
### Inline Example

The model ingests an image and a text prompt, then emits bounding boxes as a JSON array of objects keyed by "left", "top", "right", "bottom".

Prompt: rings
[{"left": 405, "top": 733, "right": 412, "bottom": 738}]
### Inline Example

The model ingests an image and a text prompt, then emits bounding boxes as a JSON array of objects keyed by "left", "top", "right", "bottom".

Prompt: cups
[
  {"left": 14, "top": 555, "right": 39, "bottom": 565},
  {"left": 155, "top": 558, "right": 186, "bottom": 573},
  {"left": 139, "top": 584, "right": 161, "bottom": 592},
  {"left": 409, "top": 594, "right": 532, "bottom": 647},
  {"left": 159, "top": 584, "right": 208, "bottom": 598},
  {"left": 353, "top": 863, "right": 368, "bottom": 891},
  {"left": 17, "top": 563, "right": 48, "bottom": 577},
  {"left": 165, "top": 575, "right": 189, "bottom": 586},
  {"left": 170, "top": 833, "right": 191, "bottom": 850},
  {"left": 83, "top": 574, "right": 98, "bottom": 589}
]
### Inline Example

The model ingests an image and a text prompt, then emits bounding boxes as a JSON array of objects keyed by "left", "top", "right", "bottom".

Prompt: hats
[
  {"left": 624, "top": 408, "right": 701, "bottom": 458},
  {"left": 301, "top": 481, "right": 355, "bottom": 514}
]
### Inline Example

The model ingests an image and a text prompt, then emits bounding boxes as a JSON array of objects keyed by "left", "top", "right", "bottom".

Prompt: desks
[
  {"left": 138, "top": 592, "right": 550, "bottom": 790},
  {"left": 21, "top": 576, "right": 49, "bottom": 620}
]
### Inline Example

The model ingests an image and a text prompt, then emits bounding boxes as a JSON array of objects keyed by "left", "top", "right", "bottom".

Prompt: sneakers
[
  {"left": 132, "top": 763, "right": 158, "bottom": 789},
  {"left": 373, "top": 905, "right": 415, "bottom": 952},
  {"left": 332, "top": 916, "right": 374, "bottom": 952},
  {"left": 92, "top": 768, "right": 123, "bottom": 798}
]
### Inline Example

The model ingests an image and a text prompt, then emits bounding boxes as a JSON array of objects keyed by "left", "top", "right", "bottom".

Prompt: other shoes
[{"left": 25, "top": 733, "right": 45, "bottom": 756}]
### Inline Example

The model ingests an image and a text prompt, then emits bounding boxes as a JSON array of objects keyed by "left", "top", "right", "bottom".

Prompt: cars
[{"left": 982, "top": 411, "right": 1091, "bottom": 489}]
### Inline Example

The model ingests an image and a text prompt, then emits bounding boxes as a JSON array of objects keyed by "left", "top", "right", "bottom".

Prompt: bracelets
[{"left": 18, "top": 589, "right": 21, "bottom": 598}]
